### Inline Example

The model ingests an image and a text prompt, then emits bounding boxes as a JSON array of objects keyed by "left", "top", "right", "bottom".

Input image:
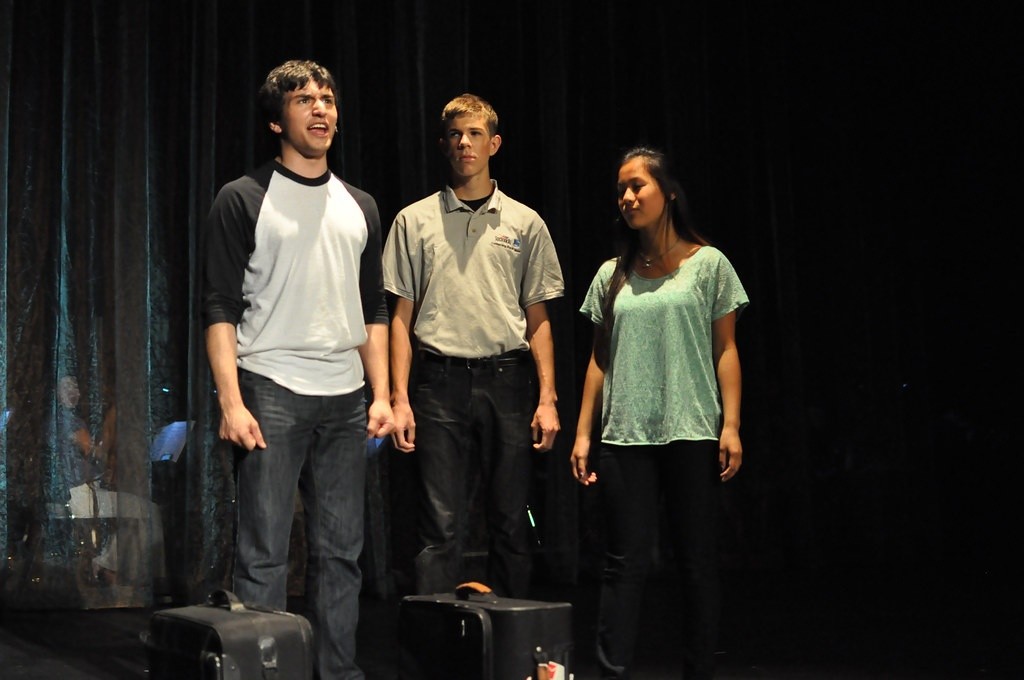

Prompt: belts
[{"left": 418, "top": 351, "right": 523, "bottom": 368}]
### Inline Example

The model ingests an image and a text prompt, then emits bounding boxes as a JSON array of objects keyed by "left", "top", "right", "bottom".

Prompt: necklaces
[{"left": 639, "top": 236, "right": 680, "bottom": 276}]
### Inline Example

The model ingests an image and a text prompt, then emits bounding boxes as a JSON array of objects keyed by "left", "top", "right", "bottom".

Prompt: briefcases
[
  {"left": 396, "top": 581, "right": 572, "bottom": 679},
  {"left": 149, "top": 587, "right": 315, "bottom": 680}
]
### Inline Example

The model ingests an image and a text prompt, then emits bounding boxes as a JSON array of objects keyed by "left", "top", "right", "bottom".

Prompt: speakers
[
  {"left": 399, "top": 592, "right": 576, "bottom": 680},
  {"left": 146, "top": 602, "right": 314, "bottom": 680}
]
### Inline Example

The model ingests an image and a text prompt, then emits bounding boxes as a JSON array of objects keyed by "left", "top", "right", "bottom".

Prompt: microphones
[
  {"left": 335, "top": 126, "right": 338, "bottom": 133},
  {"left": 616, "top": 216, "right": 621, "bottom": 222}
]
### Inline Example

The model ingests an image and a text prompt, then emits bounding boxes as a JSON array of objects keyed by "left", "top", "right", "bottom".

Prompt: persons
[
  {"left": 0, "top": 349, "right": 149, "bottom": 607},
  {"left": 203, "top": 60, "right": 394, "bottom": 680},
  {"left": 382, "top": 94, "right": 564, "bottom": 597},
  {"left": 571, "top": 148, "right": 750, "bottom": 680}
]
